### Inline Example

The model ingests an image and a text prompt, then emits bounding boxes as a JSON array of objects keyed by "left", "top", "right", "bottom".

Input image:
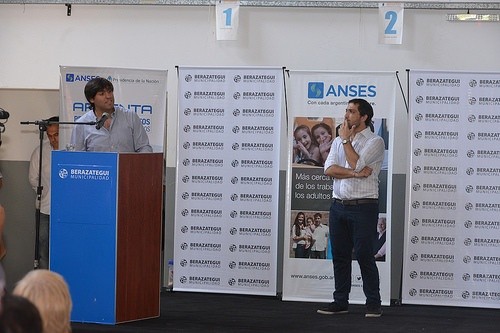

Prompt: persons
[
  {"left": 0, "top": 205, "right": 9, "bottom": 295},
  {"left": 289, "top": 209, "right": 387, "bottom": 263},
  {"left": 28, "top": 116, "right": 60, "bottom": 271},
  {"left": 0, "top": 293, "right": 44, "bottom": 333},
  {"left": 68, "top": 77, "right": 156, "bottom": 153},
  {"left": 292, "top": 116, "right": 389, "bottom": 169},
  {"left": 12, "top": 269, "right": 74, "bottom": 333},
  {"left": 316, "top": 98, "right": 386, "bottom": 319}
]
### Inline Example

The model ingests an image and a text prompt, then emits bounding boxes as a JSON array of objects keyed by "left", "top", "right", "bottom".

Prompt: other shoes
[
  {"left": 317, "top": 302, "right": 349, "bottom": 315},
  {"left": 365, "top": 306, "right": 384, "bottom": 317}
]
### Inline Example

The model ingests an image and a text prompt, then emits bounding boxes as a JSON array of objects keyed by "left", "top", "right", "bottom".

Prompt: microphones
[{"left": 96, "top": 112, "right": 110, "bottom": 130}]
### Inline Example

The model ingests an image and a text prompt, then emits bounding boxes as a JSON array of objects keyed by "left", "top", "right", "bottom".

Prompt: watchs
[{"left": 341, "top": 138, "right": 351, "bottom": 144}]
